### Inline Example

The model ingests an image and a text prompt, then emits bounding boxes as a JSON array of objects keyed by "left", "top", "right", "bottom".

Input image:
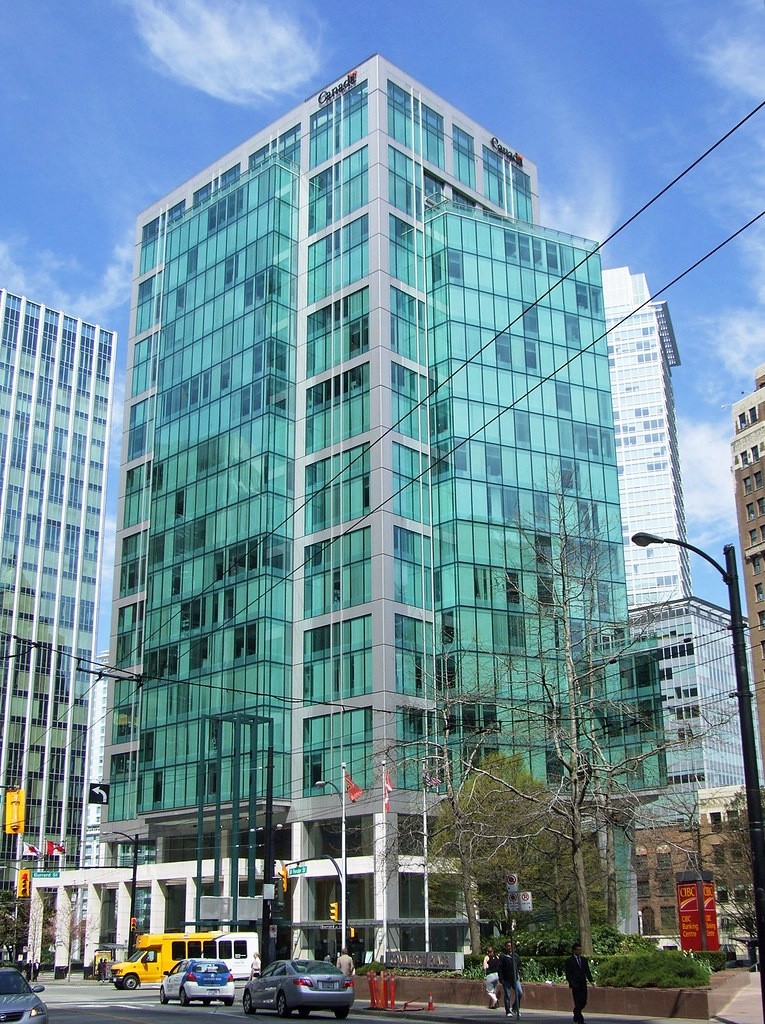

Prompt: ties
[{"left": 577, "top": 957, "right": 580, "bottom": 964}]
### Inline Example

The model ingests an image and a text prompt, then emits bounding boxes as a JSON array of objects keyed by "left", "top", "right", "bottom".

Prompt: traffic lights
[
  {"left": 18, "top": 870, "right": 29, "bottom": 898},
  {"left": 278, "top": 866, "right": 287, "bottom": 892},
  {"left": 131, "top": 918, "right": 137, "bottom": 932},
  {"left": 330, "top": 902, "right": 338, "bottom": 921}
]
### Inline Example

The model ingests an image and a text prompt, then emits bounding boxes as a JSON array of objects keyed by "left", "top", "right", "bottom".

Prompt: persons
[
  {"left": 337, "top": 948, "right": 354, "bottom": 978},
  {"left": 97, "top": 958, "right": 107, "bottom": 983},
  {"left": 498, "top": 941, "right": 524, "bottom": 1017},
  {"left": 483, "top": 947, "right": 500, "bottom": 1009},
  {"left": 324, "top": 952, "right": 332, "bottom": 962},
  {"left": 566, "top": 943, "right": 595, "bottom": 1024},
  {"left": 248, "top": 952, "right": 261, "bottom": 981},
  {"left": 27, "top": 959, "right": 40, "bottom": 982}
]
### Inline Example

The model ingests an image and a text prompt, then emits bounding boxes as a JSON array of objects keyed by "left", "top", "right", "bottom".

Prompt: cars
[
  {"left": 160, "top": 959, "right": 235, "bottom": 1007},
  {"left": 0, "top": 967, "right": 48, "bottom": 1024},
  {"left": 242, "top": 958, "right": 354, "bottom": 1019}
]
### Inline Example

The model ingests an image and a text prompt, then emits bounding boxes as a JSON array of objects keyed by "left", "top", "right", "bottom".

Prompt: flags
[
  {"left": 344, "top": 769, "right": 364, "bottom": 803},
  {"left": 383, "top": 764, "right": 393, "bottom": 812},
  {"left": 23, "top": 842, "right": 43, "bottom": 859},
  {"left": 422, "top": 771, "right": 441, "bottom": 789},
  {"left": 46, "top": 841, "right": 65, "bottom": 855}
]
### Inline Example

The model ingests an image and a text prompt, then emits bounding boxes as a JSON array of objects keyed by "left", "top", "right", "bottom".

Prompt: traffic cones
[{"left": 427, "top": 992, "right": 436, "bottom": 1011}]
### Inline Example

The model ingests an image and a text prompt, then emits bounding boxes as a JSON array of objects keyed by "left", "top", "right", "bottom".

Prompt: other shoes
[
  {"left": 513, "top": 1009, "right": 521, "bottom": 1016},
  {"left": 507, "top": 1013, "right": 513, "bottom": 1016},
  {"left": 489, "top": 1000, "right": 499, "bottom": 1009}
]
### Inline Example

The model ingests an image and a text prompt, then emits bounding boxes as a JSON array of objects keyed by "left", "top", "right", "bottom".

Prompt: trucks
[{"left": 108, "top": 931, "right": 262, "bottom": 990}]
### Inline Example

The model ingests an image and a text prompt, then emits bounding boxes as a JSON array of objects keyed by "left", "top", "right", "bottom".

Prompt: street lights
[
  {"left": 631, "top": 532, "right": 765, "bottom": 1024},
  {"left": 678, "top": 824, "right": 707, "bottom": 952},
  {"left": 102, "top": 830, "right": 139, "bottom": 959},
  {"left": 315, "top": 781, "right": 347, "bottom": 951}
]
